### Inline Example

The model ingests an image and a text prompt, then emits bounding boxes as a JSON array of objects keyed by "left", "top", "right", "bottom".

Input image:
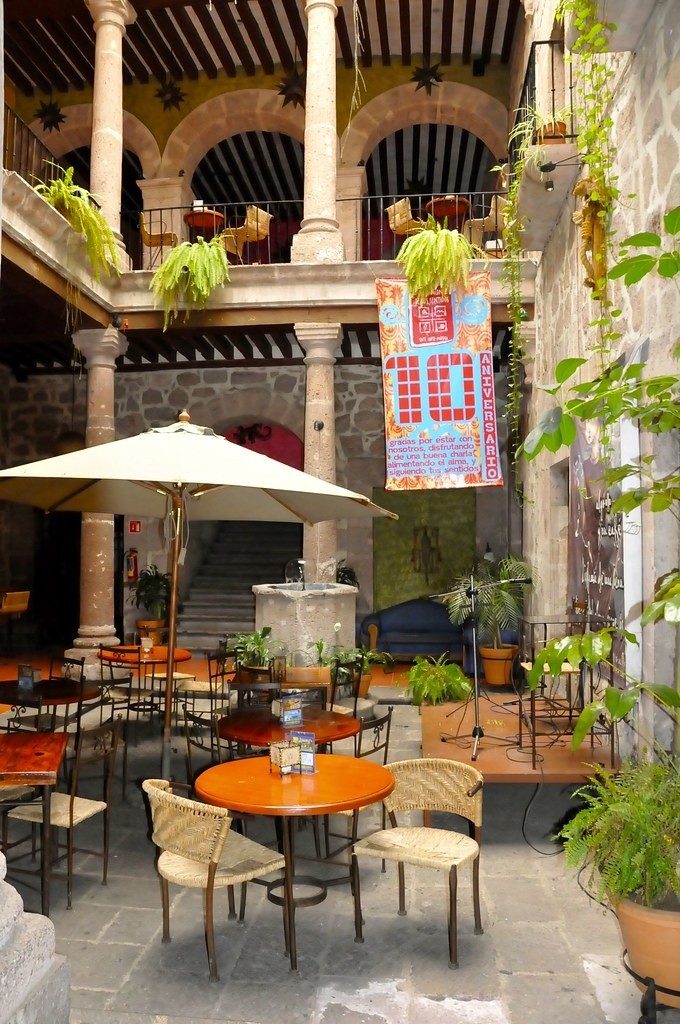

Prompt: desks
[
  {"left": 425, "top": 195, "right": 472, "bottom": 233},
  {"left": 96, "top": 644, "right": 192, "bottom": 725},
  {"left": 192, "top": 753, "right": 395, "bottom": 974},
  {"left": 0, "top": 677, "right": 103, "bottom": 791},
  {"left": 417, "top": 691, "right": 625, "bottom": 839},
  {"left": 182, "top": 206, "right": 223, "bottom": 243},
  {"left": 214, "top": 705, "right": 362, "bottom": 865}
]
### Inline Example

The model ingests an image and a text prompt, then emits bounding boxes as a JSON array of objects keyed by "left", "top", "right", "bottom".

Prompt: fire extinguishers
[{"left": 126, "top": 547, "right": 139, "bottom": 586}]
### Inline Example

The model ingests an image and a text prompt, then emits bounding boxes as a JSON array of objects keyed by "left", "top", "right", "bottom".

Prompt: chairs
[
  {"left": 140, "top": 624, "right": 196, "bottom": 728},
  {"left": 235, "top": 680, "right": 283, "bottom": 757},
  {"left": 0, "top": 589, "right": 31, "bottom": 621},
  {"left": 136, "top": 212, "right": 178, "bottom": 271},
  {"left": 8, "top": 653, "right": 86, "bottom": 743},
  {"left": 385, "top": 198, "right": 428, "bottom": 237},
  {"left": 142, "top": 777, "right": 290, "bottom": 984},
  {"left": 56, "top": 672, "right": 133, "bottom": 800},
  {"left": 97, "top": 643, "right": 156, "bottom": 733},
  {"left": 348, "top": 757, "right": 487, "bottom": 971},
  {"left": 174, "top": 648, "right": 239, "bottom": 728},
  {"left": 320, "top": 705, "right": 395, "bottom": 863},
  {"left": 461, "top": 195, "right": 514, "bottom": 250},
  {"left": 0, "top": 782, "right": 41, "bottom": 867},
  {"left": 180, "top": 703, "right": 251, "bottom": 837},
  {"left": 5, "top": 713, "right": 122, "bottom": 910},
  {"left": 217, "top": 204, "right": 275, "bottom": 265},
  {"left": 277, "top": 687, "right": 328, "bottom": 757},
  {"left": 323, "top": 652, "right": 367, "bottom": 759},
  {"left": 236, "top": 664, "right": 275, "bottom": 707}
]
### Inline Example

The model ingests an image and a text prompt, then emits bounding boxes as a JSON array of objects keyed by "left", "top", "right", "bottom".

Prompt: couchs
[{"left": 359, "top": 598, "right": 521, "bottom": 675}]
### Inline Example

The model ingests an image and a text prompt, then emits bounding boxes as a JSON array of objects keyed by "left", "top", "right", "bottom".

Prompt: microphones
[{"left": 509, "top": 577, "right": 532, "bottom": 584}]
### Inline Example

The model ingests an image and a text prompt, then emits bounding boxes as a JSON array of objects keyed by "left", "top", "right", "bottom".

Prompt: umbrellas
[{"left": 2, "top": 408, "right": 401, "bottom": 784}]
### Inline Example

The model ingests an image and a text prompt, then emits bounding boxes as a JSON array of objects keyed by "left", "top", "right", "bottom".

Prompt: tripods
[{"left": 441, "top": 587, "right": 523, "bottom": 762}]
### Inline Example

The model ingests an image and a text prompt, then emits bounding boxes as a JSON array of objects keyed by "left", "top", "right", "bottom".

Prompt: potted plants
[
  {"left": 230, "top": 626, "right": 276, "bottom": 700},
  {"left": 396, "top": 650, "right": 472, "bottom": 714},
  {"left": 552, "top": 745, "right": 678, "bottom": 1012},
  {"left": 441, "top": 555, "right": 532, "bottom": 686},
  {"left": 299, "top": 639, "right": 336, "bottom": 702},
  {"left": 126, "top": 563, "right": 183, "bottom": 647},
  {"left": 504, "top": 96, "right": 584, "bottom": 158},
  {"left": 349, "top": 645, "right": 393, "bottom": 699}
]
